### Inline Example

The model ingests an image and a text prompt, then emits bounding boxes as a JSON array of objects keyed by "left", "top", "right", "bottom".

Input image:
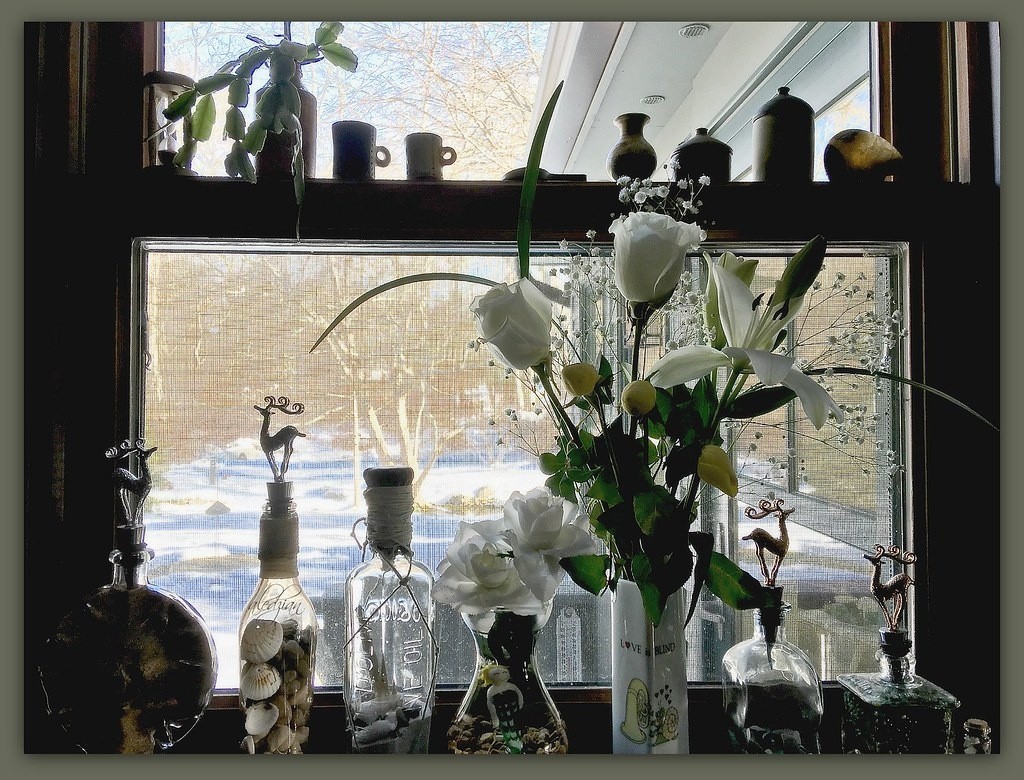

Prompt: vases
[
  {"left": 606, "top": 113, "right": 657, "bottom": 182},
  {"left": 611, "top": 578, "right": 690, "bottom": 756},
  {"left": 445, "top": 609, "right": 568, "bottom": 754}
]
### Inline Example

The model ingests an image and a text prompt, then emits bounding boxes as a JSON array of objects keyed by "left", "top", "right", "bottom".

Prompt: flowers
[
  {"left": 311, "top": 78, "right": 999, "bottom": 630},
  {"left": 430, "top": 518, "right": 557, "bottom": 682}
]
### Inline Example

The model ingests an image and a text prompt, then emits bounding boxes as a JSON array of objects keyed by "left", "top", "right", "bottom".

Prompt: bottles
[
  {"left": 38, "top": 545, "right": 215, "bottom": 755},
  {"left": 241, "top": 498, "right": 320, "bottom": 754},
  {"left": 836, "top": 635, "right": 961, "bottom": 752},
  {"left": 724, "top": 584, "right": 826, "bottom": 758},
  {"left": 606, "top": 112, "right": 658, "bottom": 182},
  {"left": 963, "top": 719, "right": 991, "bottom": 754},
  {"left": 670, "top": 127, "right": 733, "bottom": 183},
  {"left": 752, "top": 85, "right": 817, "bottom": 181},
  {"left": 339, "top": 463, "right": 440, "bottom": 749}
]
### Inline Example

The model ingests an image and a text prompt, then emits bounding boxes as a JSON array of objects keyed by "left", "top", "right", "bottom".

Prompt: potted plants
[{"left": 161, "top": 21, "right": 358, "bottom": 242}]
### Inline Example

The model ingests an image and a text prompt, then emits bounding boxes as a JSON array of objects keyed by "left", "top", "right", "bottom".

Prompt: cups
[
  {"left": 333, "top": 119, "right": 392, "bottom": 179},
  {"left": 406, "top": 132, "right": 456, "bottom": 178}
]
